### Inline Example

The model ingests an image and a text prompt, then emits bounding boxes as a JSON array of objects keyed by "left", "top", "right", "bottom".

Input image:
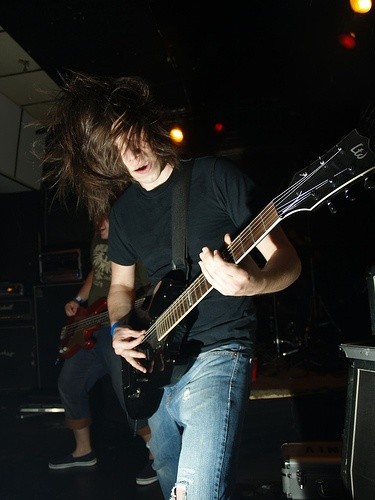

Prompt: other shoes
[
  {"left": 47, "top": 450, "right": 98, "bottom": 469},
  {"left": 135, "top": 457, "right": 160, "bottom": 486}
]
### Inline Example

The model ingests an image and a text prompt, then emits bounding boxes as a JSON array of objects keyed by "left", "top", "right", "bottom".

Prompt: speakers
[
  {"left": 341, "top": 358, "right": 375, "bottom": 500},
  {"left": 0, "top": 282, "right": 87, "bottom": 393}
]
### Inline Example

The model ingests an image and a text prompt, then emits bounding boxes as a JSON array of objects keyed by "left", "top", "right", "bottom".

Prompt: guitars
[
  {"left": 57, "top": 297, "right": 145, "bottom": 357},
  {"left": 119, "top": 129, "right": 375, "bottom": 419}
]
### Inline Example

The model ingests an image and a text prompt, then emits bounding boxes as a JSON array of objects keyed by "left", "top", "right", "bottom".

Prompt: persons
[
  {"left": 48, "top": 214, "right": 158, "bottom": 485},
  {"left": 24, "top": 67, "right": 301, "bottom": 500}
]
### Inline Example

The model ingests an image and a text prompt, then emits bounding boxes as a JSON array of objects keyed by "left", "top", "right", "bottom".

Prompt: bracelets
[
  {"left": 73, "top": 295, "right": 84, "bottom": 304},
  {"left": 111, "top": 321, "right": 128, "bottom": 337}
]
